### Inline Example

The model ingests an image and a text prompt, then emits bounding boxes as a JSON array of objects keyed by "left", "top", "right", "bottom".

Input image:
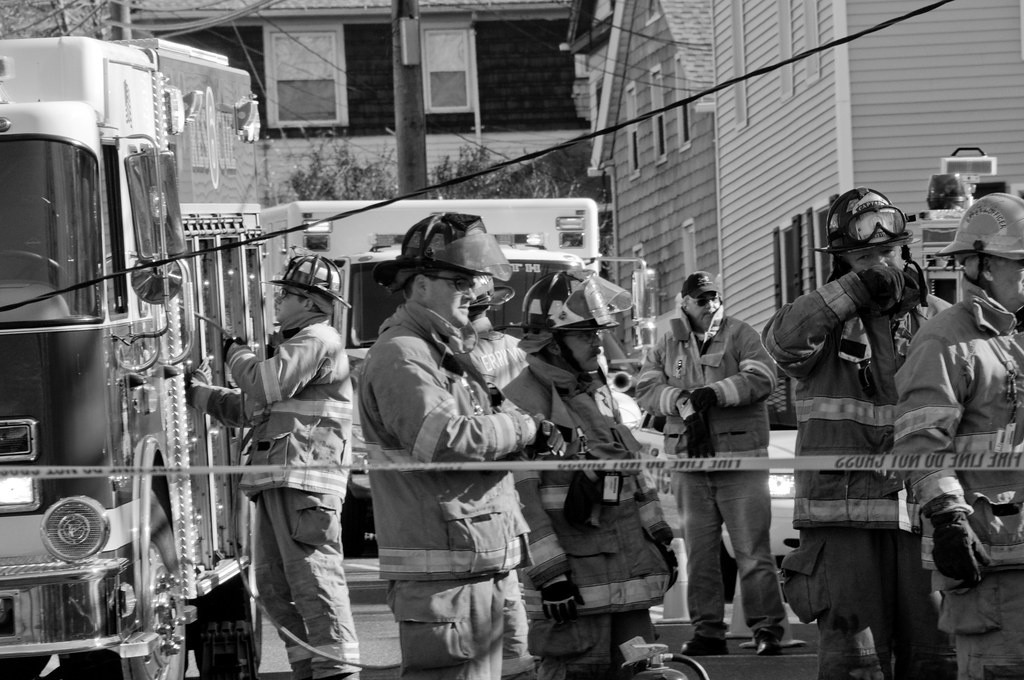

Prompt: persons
[
  {"left": 637, "top": 271, "right": 788, "bottom": 657},
  {"left": 760, "top": 186, "right": 959, "bottom": 680},
  {"left": 186, "top": 254, "right": 364, "bottom": 680},
  {"left": 502, "top": 268, "right": 680, "bottom": 680},
  {"left": 357, "top": 210, "right": 568, "bottom": 680},
  {"left": 892, "top": 188, "right": 1024, "bottom": 680},
  {"left": 468, "top": 274, "right": 529, "bottom": 389}
]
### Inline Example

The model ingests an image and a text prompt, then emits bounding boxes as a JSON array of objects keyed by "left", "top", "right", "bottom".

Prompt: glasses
[
  {"left": 559, "top": 327, "right": 607, "bottom": 344},
  {"left": 686, "top": 295, "right": 721, "bottom": 307},
  {"left": 835, "top": 205, "right": 910, "bottom": 248},
  {"left": 277, "top": 288, "right": 320, "bottom": 312},
  {"left": 420, "top": 271, "right": 480, "bottom": 293}
]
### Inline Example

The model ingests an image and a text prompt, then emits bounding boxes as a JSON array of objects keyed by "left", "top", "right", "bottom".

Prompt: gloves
[
  {"left": 184, "top": 376, "right": 201, "bottom": 406},
  {"left": 930, "top": 511, "right": 993, "bottom": 582},
  {"left": 855, "top": 265, "right": 906, "bottom": 307},
  {"left": 223, "top": 336, "right": 246, "bottom": 360},
  {"left": 691, "top": 387, "right": 718, "bottom": 412},
  {"left": 682, "top": 413, "right": 716, "bottom": 459},
  {"left": 540, "top": 574, "right": 585, "bottom": 624},
  {"left": 652, "top": 530, "right": 678, "bottom": 592},
  {"left": 519, "top": 407, "right": 569, "bottom": 457}
]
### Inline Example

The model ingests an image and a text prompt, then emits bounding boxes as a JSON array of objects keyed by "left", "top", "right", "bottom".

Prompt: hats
[{"left": 680, "top": 271, "right": 722, "bottom": 301}]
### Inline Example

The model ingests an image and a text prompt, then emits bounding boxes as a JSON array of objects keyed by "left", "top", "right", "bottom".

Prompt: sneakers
[
  {"left": 680, "top": 634, "right": 729, "bottom": 657},
  {"left": 757, "top": 635, "right": 784, "bottom": 655}
]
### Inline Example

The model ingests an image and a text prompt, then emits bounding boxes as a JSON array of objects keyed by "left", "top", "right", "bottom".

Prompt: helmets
[
  {"left": 503, "top": 270, "right": 620, "bottom": 333},
  {"left": 269, "top": 252, "right": 352, "bottom": 308},
  {"left": 396, "top": 212, "right": 502, "bottom": 275},
  {"left": 372, "top": 251, "right": 438, "bottom": 288},
  {"left": 463, "top": 267, "right": 516, "bottom": 305},
  {"left": 936, "top": 193, "right": 1024, "bottom": 260},
  {"left": 813, "top": 187, "right": 914, "bottom": 253}
]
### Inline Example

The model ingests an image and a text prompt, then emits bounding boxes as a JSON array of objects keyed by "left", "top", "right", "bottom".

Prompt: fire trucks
[
  {"left": 769, "top": 146, "right": 1023, "bottom": 331},
  {"left": 252, "top": 196, "right": 658, "bottom": 532},
  {"left": 0, "top": 36, "right": 273, "bottom": 678}
]
